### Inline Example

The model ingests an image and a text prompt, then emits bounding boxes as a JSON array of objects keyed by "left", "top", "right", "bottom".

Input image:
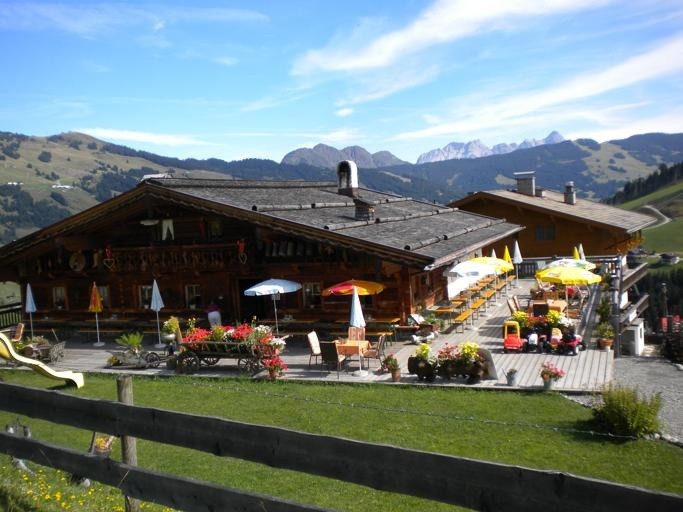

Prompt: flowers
[
  {"left": 377, "top": 353, "right": 399, "bottom": 374},
  {"left": 179, "top": 316, "right": 288, "bottom": 373},
  {"left": 503, "top": 310, "right": 562, "bottom": 334},
  {"left": 413, "top": 341, "right": 567, "bottom": 386}
]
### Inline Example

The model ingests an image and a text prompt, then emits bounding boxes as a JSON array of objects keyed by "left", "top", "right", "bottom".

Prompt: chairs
[
  {"left": 307, "top": 327, "right": 386, "bottom": 379},
  {"left": 506, "top": 276, "right": 591, "bottom": 320},
  {"left": 9, "top": 323, "right": 24, "bottom": 342},
  {"left": 23, "top": 346, "right": 36, "bottom": 359}
]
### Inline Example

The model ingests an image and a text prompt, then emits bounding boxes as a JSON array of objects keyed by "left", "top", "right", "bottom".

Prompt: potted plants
[
  {"left": 115, "top": 332, "right": 144, "bottom": 353},
  {"left": 591, "top": 305, "right": 616, "bottom": 350},
  {"left": 160, "top": 317, "right": 179, "bottom": 342}
]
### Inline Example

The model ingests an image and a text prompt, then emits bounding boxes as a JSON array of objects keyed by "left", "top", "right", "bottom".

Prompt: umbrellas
[
  {"left": 243, "top": 277, "right": 303, "bottom": 337},
  {"left": 26, "top": 283, "right": 37, "bottom": 340},
  {"left": 446, "top": 240, "right": 604, "bottom": 325},
  {"left": 349, "top": 286, "right": 366, "bottom": 372},
  {"left": 87, "top": 281, "right": 104, "bottom": 343},
  {"left": 321, "top": 277, "right": 387, "bottom": 340},
  {"left": 150, "top": 279, "right": 164, "bottom": 344}
]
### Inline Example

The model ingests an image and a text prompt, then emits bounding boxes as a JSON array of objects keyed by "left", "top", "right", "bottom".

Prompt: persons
[{"left": 207, "top": 300, "right": 222, "bottom": 329}]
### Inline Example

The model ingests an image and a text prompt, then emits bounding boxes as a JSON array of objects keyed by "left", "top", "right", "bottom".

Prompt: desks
[
  {"left": 336, "top": 318, "right": 401, "bottom": 343},
  {"left": 426, "top": 275, "right": 499, "bottom": 334}
]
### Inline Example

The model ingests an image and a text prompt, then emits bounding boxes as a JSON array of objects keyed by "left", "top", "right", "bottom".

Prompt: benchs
[
  {"left": 273, "top": 331, "right": 311, "bottom": 337},
  {"left": 329, "top": 331, "right": 394, "bottom": 346},
  {"left": 495, "top": 276, "right": 516, "bottom": 298},
  {"left": 23, "top": 328, "right": 163, "bottom": 334}
]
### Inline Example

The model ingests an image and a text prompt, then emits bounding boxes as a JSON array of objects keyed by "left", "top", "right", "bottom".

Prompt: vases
[
  {"left": 543, "top": 379, "right": 553, "bottom": 389},
  {"left": 269, "top": 370, "right": 280, "bottom": 378},
  {"left": 392, "top": 369, "right": 401, "bottom": 382}
]
